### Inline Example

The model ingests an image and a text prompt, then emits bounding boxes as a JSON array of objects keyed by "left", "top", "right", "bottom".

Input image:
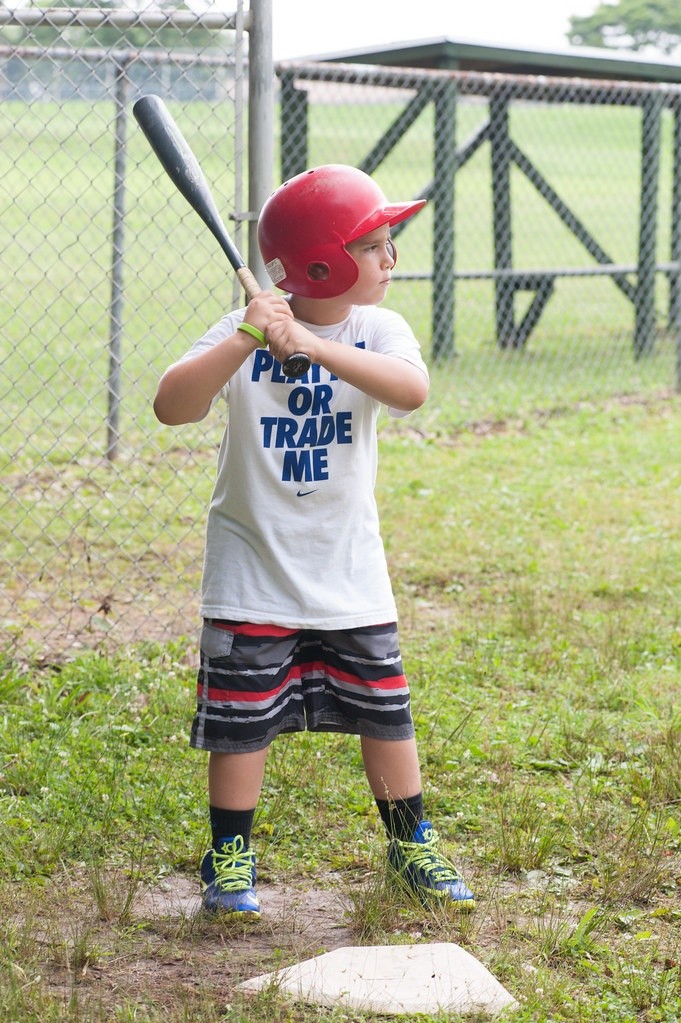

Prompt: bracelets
[{"left": 238, "top": 322, "right": 269, "bottom": 349}]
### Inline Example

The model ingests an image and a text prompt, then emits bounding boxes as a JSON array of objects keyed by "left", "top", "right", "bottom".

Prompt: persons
[{"left": 153, "top": 165, "right": 476, "bottom": 922}]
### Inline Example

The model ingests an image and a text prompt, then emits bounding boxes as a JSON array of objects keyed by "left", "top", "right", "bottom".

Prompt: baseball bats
[{"left": 132, "top": 94, "right": 311, "bottom": 380}]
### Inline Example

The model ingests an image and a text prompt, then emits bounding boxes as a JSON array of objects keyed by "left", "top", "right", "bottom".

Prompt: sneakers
[
  {"left": 199, "top": 837, "right": 263, "bottom": 923},
  {"left": 386, "top": 820, "right": 477, "bottom": 914}
]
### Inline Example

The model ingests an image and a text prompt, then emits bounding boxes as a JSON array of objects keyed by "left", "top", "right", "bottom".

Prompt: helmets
[{"left": 257, "top": 164, "right": 427, "bottom": 301}]
